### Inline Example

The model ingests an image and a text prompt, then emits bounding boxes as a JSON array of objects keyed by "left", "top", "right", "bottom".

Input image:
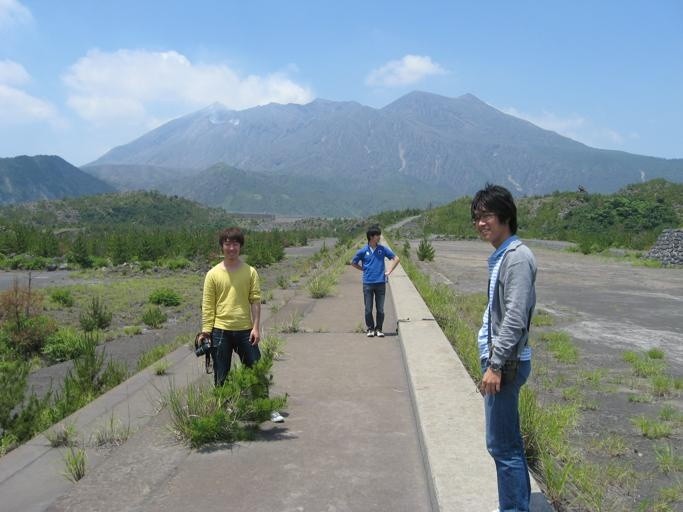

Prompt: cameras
[{"left": 194, "top": 337, "right": 213, "bottom": 357}]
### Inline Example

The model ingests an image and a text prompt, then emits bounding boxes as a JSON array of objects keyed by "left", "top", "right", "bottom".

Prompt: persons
[
  {"left": 467, "top": 182, "right": 540, "bottom": 512},
  {"left": 196, "top": 225, "right": 286, "bottom": 424},
  {"left": 350, "top": 226, "right": 399, "bottom": 338}
]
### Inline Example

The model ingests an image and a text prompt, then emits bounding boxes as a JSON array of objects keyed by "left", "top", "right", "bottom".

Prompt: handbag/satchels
[{"left": 487, "top": 357, "right": 518, "bottom": 385}]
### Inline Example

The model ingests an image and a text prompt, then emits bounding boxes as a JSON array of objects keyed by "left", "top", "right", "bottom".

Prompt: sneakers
[
  {"left": 263, "top": 410, "right": 285, "bottom": 423},
  {"left": 366, "top": 329, "right": 384, "bottom": 337}
]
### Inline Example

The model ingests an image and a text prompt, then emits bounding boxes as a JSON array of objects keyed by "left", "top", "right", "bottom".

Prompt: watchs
[{"left": 488, "top": 360, "right": 504, "bottom": 373}]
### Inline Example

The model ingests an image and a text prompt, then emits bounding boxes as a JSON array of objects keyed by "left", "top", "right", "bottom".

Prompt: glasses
[{"left": 471, "top": 213, "right": 496, "bottom": 225}]
